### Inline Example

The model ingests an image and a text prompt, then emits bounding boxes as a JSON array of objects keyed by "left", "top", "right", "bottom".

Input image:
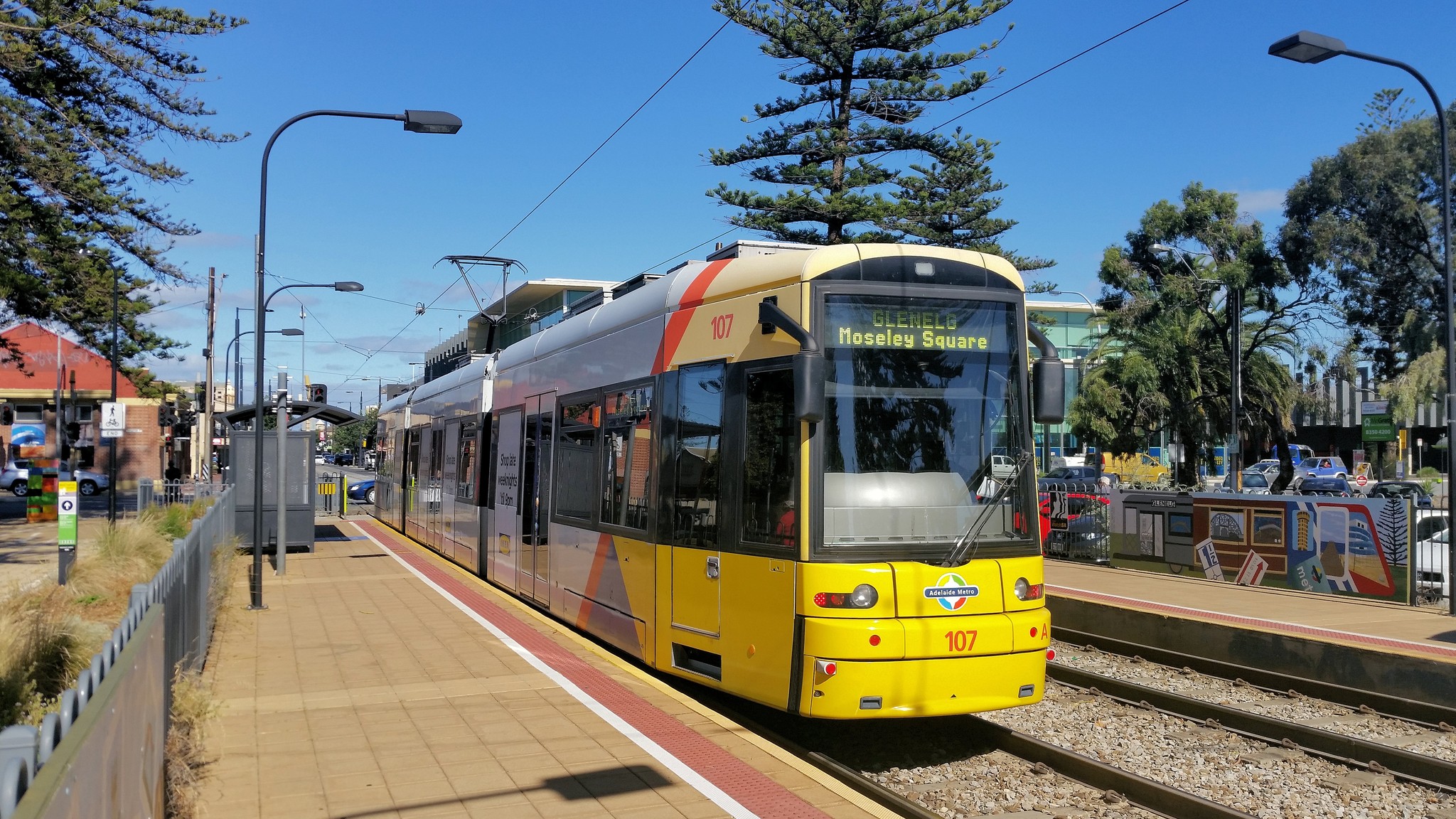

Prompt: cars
[
  {"left": 347, "top": 479, "right": 375, "bottom": 505},
  {"left": 315, "top": 455, "right": 325, "bottom": 465},
  {"left": 321, "top": 451, "right": 357, "bottom": 467},
  {"left": 992, "top": 442, "right": 1450, "bottom": 609}
]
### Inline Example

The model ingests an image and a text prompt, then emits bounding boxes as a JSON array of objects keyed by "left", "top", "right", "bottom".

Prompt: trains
[{"left": 374, "top": 241, "right": 1054, "bottom": 719}]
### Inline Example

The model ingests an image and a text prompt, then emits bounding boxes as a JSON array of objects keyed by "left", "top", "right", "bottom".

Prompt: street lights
[
  {"left": 248, "top": 110, "right": 473, "bottom": 608},
  {"left": 439, "top": 327, "right": 443, "bottom": 344},
  {"left": 1049, "top": 289, "right": 1103, "bottom": 484},
  {"left": 337, "top": 402, "right": 352, "bottom": 412},
  {"left": 409, "top": 363, "right": 433, "bottom": 382},
  {"left": 239, "top": 357, "right": 267, "bottom": 407},
  {"left": 481, "top": 298, "right": 487, "bottom": 310},
  {"left": 1268, "top": 30, "right": 1456, "bottom": 614},
  {"left": 234, "top": 307, "right": 275, "bottom": 408},
  {"left": 361, "top": 379, "right": 381, "bottom": 410},
  {"left": 345, "top": 391, "right": 363, "bottom": 468},
  {"left": 458, "top": 314, "right": 462, "bottom": 332},
  {"left": 78, "top": 247, "right": 119, "bottom": 523},
  {"left": 222, "top": 328, "right": 304, "bottom": 495},
  {"left": 1148, "top": 243, "right": 1243, "bottom": 493}
]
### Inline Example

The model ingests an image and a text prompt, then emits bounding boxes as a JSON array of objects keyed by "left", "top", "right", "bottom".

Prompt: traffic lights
[
  {"left": 158, "top": 405, "right": 177, "bottom": 427},
  {"left": 180, "top": 409, "right": 196, "bottom": 422},
  {"left": 1, "top": 403, "right": 14, "bottom": 426},
  {"left": 165, "top": 436, "right": 172, "bottom": 442},
  {"left": 310, "top": 383, "right": 327, "bottom": 404}
]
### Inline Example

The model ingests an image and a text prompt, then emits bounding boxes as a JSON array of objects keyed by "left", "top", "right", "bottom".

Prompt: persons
[
  {"left": 164, "top": 460, "right": 182, "bottom": 503},
  {"left": 1323, "top": 461, "right": 1330, "bottom": 468}
]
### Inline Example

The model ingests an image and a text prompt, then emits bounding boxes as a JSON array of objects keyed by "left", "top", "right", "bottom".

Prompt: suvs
[
  {"left": 364, "top": 453, "right": 376, "bottom": 472},
  {"left": 0, "top": 458, "right": 110, "bottom": 497}
]
